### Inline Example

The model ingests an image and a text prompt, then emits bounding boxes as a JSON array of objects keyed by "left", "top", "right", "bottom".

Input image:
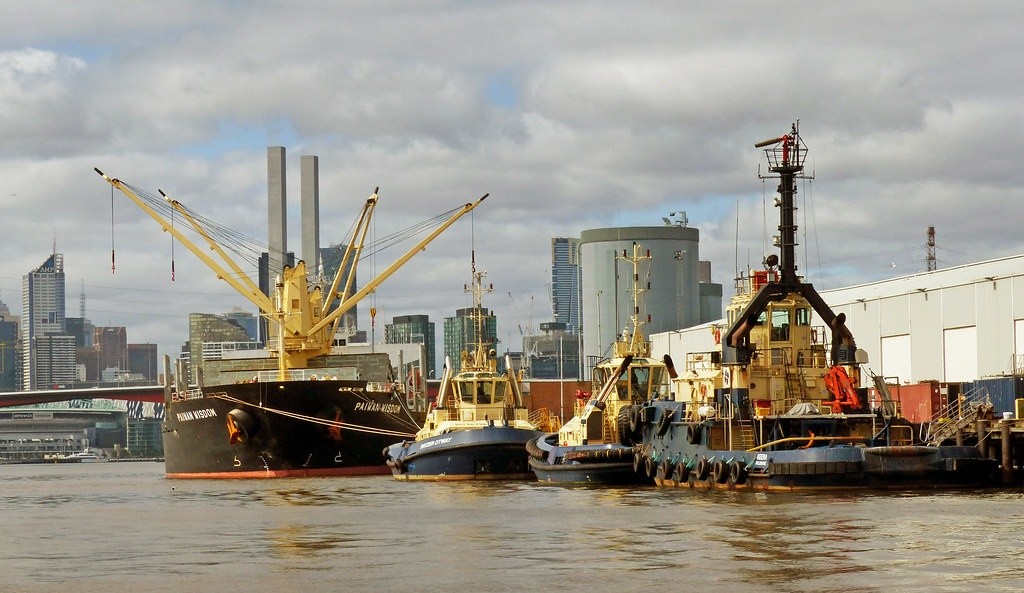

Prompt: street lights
[
  {"left": 144, "top": 341, "right": 150, "bottom": 385},
  {"left": 557, "top": 330, "right": 564, "bottom": 429},
  {"left": 591, "top": 289, "right": 604, "bottom": 362},
  {"left": 32, "top": 337, "right": 37, "bottom": 391}
]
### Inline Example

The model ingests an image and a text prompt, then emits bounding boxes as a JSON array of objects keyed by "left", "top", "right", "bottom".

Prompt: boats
[
  {"left": 93, "top": 166, "right": 491, "bottom": 478},
  {"left": 382, "top": 270, "right": 551, "bottom": 480},
  {"left": 526, "top": 245, "right": 676, "bottom": 485},
  {"left": 618, "top": 115, "right": 997, "bottom": 494},
  {"left": 76, "top": 445, "right": 109, "bottom": 463}
]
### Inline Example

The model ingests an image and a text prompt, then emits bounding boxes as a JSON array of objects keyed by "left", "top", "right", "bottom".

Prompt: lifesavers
[
  {"left": 731, "top": 461, "right": 745, "bottom": 484},
  {"left": 662, "top": 458, "right": 674, "bottom": 479},
  {"left": 654, "top": 408, "right": 673, "bottom": 437},
  {"left": 700, "top": 383, "right": 706, "bottom": 397},
  {"left": 696, "top": 459, "right": 711, "bottom": 481},
  {"left": 675, "top": 462, "right": 689, "bottom": 482},
  {"left": 394, "top": 460, "right": 405, "bottom": 475},
  {"left": 714, "top": 329, "right": 720, "bottom": 344},
  {"left": 633, "top": 452, "right": 643, "bottom": 473},
  {"left": 616, "top": 404, "right": 642, "bottom": 447},
  {"left": 712, "top": 460, "right": 729, "bottom": 483},
  {"left": 645, "top": 457, "right": 657, "bottom": 478},
  {"left": 686, "top": 423, "right": 702, "bottom": 445}
]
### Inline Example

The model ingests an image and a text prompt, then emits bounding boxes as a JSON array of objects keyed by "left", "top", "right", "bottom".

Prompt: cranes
[{"left": 507, "top": 291, "right": 538, "bottom": 378}]
